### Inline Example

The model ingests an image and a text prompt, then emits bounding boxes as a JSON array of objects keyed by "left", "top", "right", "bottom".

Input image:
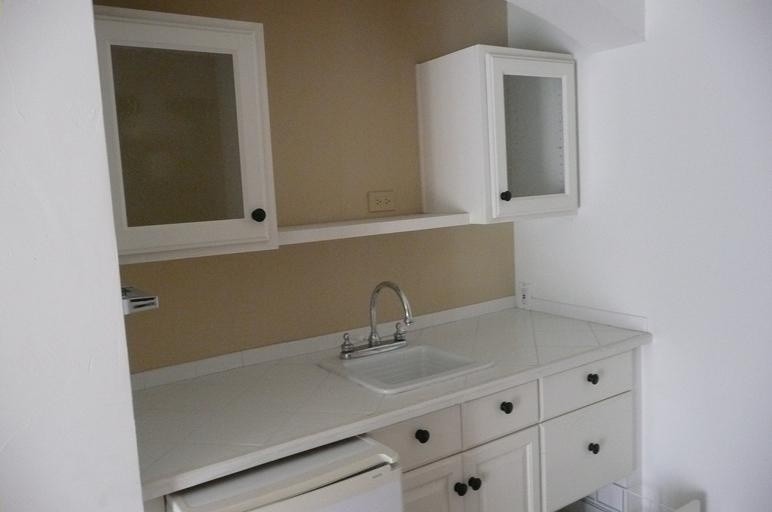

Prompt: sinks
[{"left": 315, "top": 343, "right": 495, "bottom": 396}]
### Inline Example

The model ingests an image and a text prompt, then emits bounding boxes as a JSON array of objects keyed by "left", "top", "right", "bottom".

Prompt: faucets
[{"left": 369, "top": 281, "right": 415, "bottom": 347}]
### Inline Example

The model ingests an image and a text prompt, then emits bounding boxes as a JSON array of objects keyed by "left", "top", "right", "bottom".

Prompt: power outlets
[{"left": 367, "top": 190, "right": 396, "bottom": 212}]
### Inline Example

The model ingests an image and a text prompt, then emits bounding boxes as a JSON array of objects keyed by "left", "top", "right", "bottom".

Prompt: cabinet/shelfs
[
  {"left": 360, "top": 379, "right": 543, "bottom": 512},
  {"left": 415, "top": 41, "right": 580, "bottom": 227},
  {"left": 538, "top": 347, "right": 635, "bottom": 510},
  {"left": 92, "top": 4, "right": 280, "bottom": 268}
]
